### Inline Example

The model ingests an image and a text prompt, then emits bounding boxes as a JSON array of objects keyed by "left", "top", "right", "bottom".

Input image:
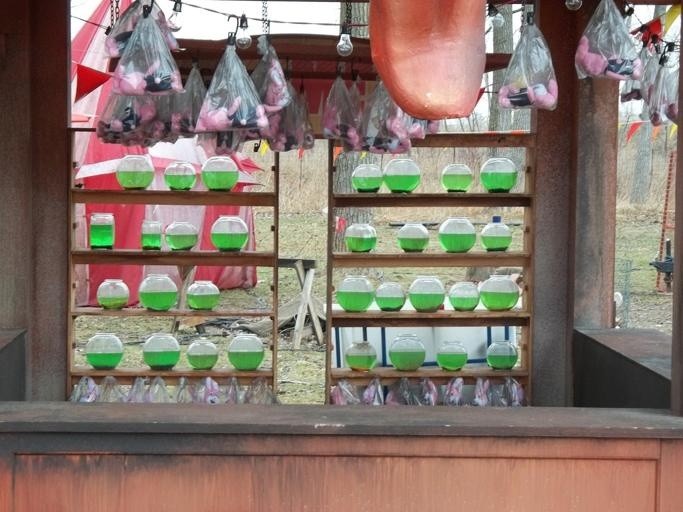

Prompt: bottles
[
  {"left": 141, "top": 220, "right": 162, "bottom": 250},
  {"left": 89, "top": 212, "right": 116, "bottom": 249}
]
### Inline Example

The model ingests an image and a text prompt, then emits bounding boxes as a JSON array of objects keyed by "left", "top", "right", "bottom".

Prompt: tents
[{"left": 68, "top": 0, "right": 260, "bottom": 308}]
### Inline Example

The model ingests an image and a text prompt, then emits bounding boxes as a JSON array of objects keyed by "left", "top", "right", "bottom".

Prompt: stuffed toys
[
  {"left": 203, "top": 377, "right": 222, "bottom": 404},
  {"left": 573, "top": 35, "right": 678, "bottom": 128},
  {"left": 331, "top": 379, "right": 526, "bottom": 406},
  {"left": 319, "top": 93, "right": 440, "bottom": 153},
  {"left": 96, "top": 9, "right": 315, "bottom": 153},
  {"left": 78, "top": 378, "right": 98, "bottom": 402},
  {"left": 498, "top": 77, "right": 558, "bottom": 114}
]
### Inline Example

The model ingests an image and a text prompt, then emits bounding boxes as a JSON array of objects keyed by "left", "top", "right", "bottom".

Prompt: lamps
[
  {"left": 485, "top": 1, "right": 505, "bottom": 30},
  {"left": 235, "top": 14, "right": 251, "bottom": 50},
  {"left": 335, "top": 22, "right": 353, "bottom": 58},
  {"left": 166, "top": 0, "right": 185, "bottom": 31}
]
